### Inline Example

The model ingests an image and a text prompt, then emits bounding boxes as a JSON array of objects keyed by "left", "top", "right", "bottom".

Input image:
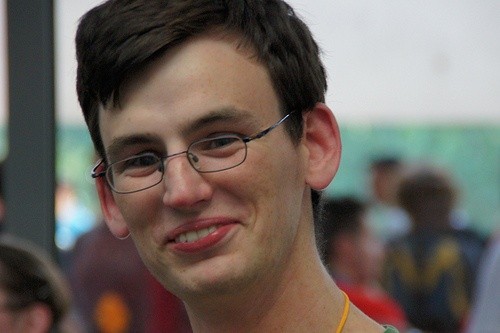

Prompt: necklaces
[{"left": 335, "top": 288, "right": 350, "bottom": 333}]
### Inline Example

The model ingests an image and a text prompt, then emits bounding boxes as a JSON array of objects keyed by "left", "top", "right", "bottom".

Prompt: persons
[
  {"left": 321, "top": 158, "right": 500, "bottom": 333},
  {"left": 0, "top": 162, "right": 193, "bottom": 333},
  {"left": 75, "top": 0, "right": 399, "bottom": 333}
]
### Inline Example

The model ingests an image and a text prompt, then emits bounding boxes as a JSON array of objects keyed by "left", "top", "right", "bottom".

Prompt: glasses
[{"left": 91, "top": 108, "right": 295, "bottom": 196}]
